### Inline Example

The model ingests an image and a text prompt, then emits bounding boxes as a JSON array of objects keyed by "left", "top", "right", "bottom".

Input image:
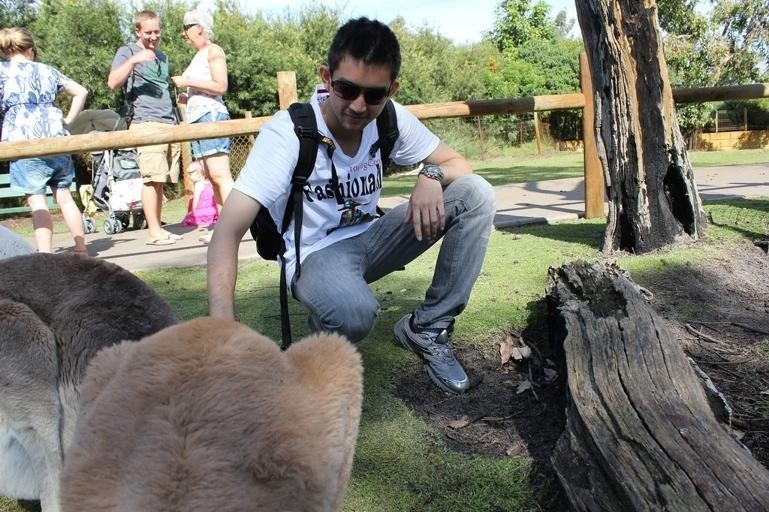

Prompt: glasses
[
  {"left": 183, "top": 24, "right": 199, "bottom": 30},
  {"left": 330, "top": 76, "right": 393, "bottom": 105}
]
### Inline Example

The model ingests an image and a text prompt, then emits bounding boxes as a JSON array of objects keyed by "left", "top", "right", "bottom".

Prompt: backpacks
[{"left": 250, "top": 205, "right": 287, "bottom": 260}]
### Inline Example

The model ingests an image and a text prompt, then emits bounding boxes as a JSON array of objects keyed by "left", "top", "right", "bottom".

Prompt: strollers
[{"left": 81, "top": 116, "right": 149, "bottom": 236}]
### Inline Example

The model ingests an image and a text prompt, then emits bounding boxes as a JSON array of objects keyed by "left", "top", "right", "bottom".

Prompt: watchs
[{"left": 418, "top": 164, "right": 444, "bottom": 181}]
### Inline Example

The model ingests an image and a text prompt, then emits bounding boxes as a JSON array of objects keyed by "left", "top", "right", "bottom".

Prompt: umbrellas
[{"left": 63, "top": 109, "right": 120, "bottom": 135}]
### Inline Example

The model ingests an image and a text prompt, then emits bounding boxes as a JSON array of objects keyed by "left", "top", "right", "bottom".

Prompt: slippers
[{"left": 145, "top": 232, "right": 184, "bottom": 245}]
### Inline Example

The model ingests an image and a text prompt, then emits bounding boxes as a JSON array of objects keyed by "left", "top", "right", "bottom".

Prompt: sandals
[{"left": 197, "top": 227, "right": 211, "bottom": 244}]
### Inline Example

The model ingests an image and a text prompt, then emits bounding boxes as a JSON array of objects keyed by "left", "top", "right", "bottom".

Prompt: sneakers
[{"left": 393, "top": 313, "right": 469, "bottom": 395}]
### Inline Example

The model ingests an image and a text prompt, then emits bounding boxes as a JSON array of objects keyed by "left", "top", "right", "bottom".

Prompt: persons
[
  {"left": 1, "top": 26, "right": 88, "bottom": 256},
  {"left": 171, "top": 11, "right": 238, "bottom": 215},
  {"left": 107, "top": 10, "right": 185, "bottom": 246},
  {"left": 180, "top": 161, "right": 219, "bottom": 244},
  {"left": 70, "top": 154, "right": 98, "bottom": 218},
  {"left": 205, "top": 17, "right": 497, "bottom": 397}
]
